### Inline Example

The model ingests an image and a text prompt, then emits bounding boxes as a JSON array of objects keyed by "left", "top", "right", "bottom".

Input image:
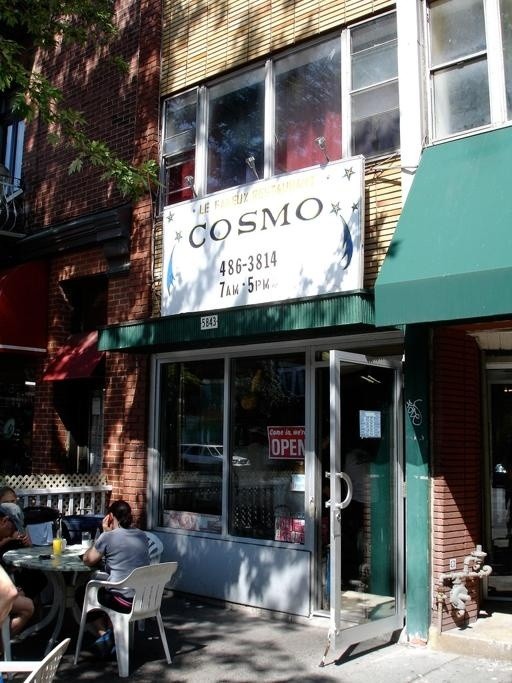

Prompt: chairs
[
  {"left": 0, "top": 638, "right": 73, "bottom": 683},
  {"left": 73, "top": 560, "right": 180, "bottom": 678}
]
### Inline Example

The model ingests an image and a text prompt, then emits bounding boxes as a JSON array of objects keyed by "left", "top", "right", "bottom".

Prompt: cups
[
  {"left": 81, "top": 531, "right": 92, "bottom": 548},
  {"left": 53, "top": 538, "right": 62, "bottom": 556}
]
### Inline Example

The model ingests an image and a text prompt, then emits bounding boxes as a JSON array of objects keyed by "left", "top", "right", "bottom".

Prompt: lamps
[
  {"left": 186, "top": 175, "right": 197, "bottom": 198},
  {"left": 313, "top": 136, "right": 332, "bottom": 162},
  {"left": 244, "top": 157, "right": 260, "bottom": 179}
]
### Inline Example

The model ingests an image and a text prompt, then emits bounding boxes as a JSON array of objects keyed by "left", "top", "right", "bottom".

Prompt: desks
[{"left": 6, "top": 544, "right": 101, "bottom": 657}]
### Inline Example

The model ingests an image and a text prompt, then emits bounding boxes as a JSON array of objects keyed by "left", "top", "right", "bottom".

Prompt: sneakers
[{"left": 95, "top": 630, "right": 116, "bottom": 658}]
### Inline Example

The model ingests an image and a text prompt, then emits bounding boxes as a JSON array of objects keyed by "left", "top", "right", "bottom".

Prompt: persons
[
  {"left": 228, "top": 415, "right": 386, "bottom": 592},
  {"left": 72, "top": 498, "right": 152, "bottom": 655},
  {"left": 0, "top": 485, "right": 34, "bottom": 598},
  {"left": 0, "top": 562, "right": 21, "bottom": 628},
  {"left": 0, "top": 500, "right": 38, "bottom": 679}
]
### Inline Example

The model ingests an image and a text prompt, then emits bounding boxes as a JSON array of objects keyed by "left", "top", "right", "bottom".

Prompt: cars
[{"left": 180, "top": 443, "right": 251, "bottom": 474}]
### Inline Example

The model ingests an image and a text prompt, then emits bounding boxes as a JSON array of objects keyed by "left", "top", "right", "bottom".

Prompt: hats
[{"left": 0, "top": 502, "right": 25, "bottom": 534}]
